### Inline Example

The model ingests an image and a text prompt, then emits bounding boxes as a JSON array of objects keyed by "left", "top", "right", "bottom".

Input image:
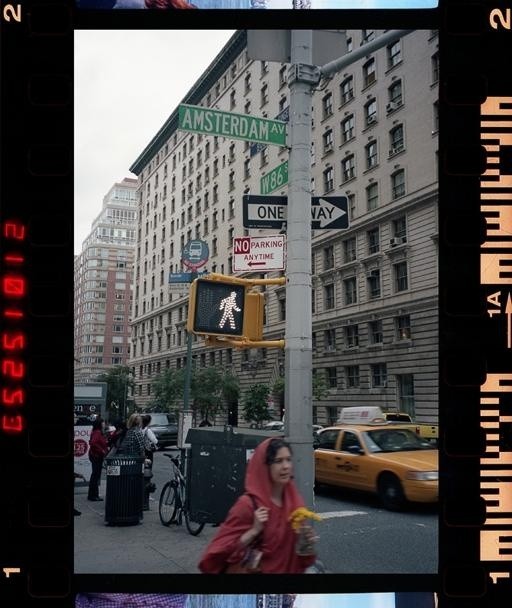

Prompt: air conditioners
[
  {"left": 389, "top": 149, "right": 398, "bottom": 156},
  {"left": 384, "top": 102, "right": 397, "bottom": 112},
  {"left": 390, "top": 238, "right": 402, "bottom": 246},
  {"left": 367, "top": 115, "right": 376, "bottom": 124}
]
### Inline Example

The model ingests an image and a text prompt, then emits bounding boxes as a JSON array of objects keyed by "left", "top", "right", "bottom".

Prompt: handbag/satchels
[
  {"left": 142, "top": 434, "right": 160, "bottom": 452},
  {"left": 225, "top": 547, "right": 263, "bottom": 574}
]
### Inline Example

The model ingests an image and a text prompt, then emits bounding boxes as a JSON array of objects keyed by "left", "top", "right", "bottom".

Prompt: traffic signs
[
  {"left": 232, "top": 234, "right": 285, "bottom": 272},
  {"left": 167, "top": 273, "right": 214, "bottom": 293},
  {"left": 178, "top": 104, "right": 286, "bottom": 148},
  {"left": 260, "top": 161, "right": 288, "bottom": 196},
  {"left": 181, "top": 239, "right": 209, "bottom": 271}
]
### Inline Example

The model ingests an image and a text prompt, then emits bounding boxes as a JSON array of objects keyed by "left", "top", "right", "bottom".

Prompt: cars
[
  {"left": 264, "top": 421, "right": 323, "bottom": 433},
  {"left": 139, "top": 413, "right": 178, "bottom": 450},
  {"left": 314, "top": 406, "right": 439, "bottom": 511}
]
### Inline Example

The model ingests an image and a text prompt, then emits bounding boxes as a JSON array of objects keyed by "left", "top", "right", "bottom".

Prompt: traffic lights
[{"left": 187, "top": 280, "right": 245, "bottom": 337}]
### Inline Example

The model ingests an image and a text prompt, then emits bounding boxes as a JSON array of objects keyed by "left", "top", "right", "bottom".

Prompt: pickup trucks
[{"left": 383, "top": 412, "right": 438, "bottom": 439}]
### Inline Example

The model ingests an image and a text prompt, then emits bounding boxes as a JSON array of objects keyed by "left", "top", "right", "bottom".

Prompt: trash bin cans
[{"left": 104, "top": 455, "right": 146, "bottom": 526}]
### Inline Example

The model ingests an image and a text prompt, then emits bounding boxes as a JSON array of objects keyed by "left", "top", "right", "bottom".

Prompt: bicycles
[{"left": 158, "top": 453, "right": 206, "bottom": 536}]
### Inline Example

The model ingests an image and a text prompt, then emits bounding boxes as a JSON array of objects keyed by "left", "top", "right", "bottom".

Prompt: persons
[
  {"left": 72, "top": 412, "right": 81, "bottom": 515},
  {"left": 198, "top": 436, "right": 322, "bottom": 574},
  {"left": 88, "top": 419, "right": 108, "bottom": 502},
  {"left": 198, "top": 418, "right": 212, "bottom": 427},
  {"left": 141, "top": 414, "right": 160, "bottom": 473},
  {"left": 116, "top": 412, "right": 152, "bottom": 466}
]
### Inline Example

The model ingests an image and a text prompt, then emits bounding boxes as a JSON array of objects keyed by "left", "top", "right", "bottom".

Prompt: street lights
[{"left": 242, "top": 194, "right": 350, "bottom": 231}]
